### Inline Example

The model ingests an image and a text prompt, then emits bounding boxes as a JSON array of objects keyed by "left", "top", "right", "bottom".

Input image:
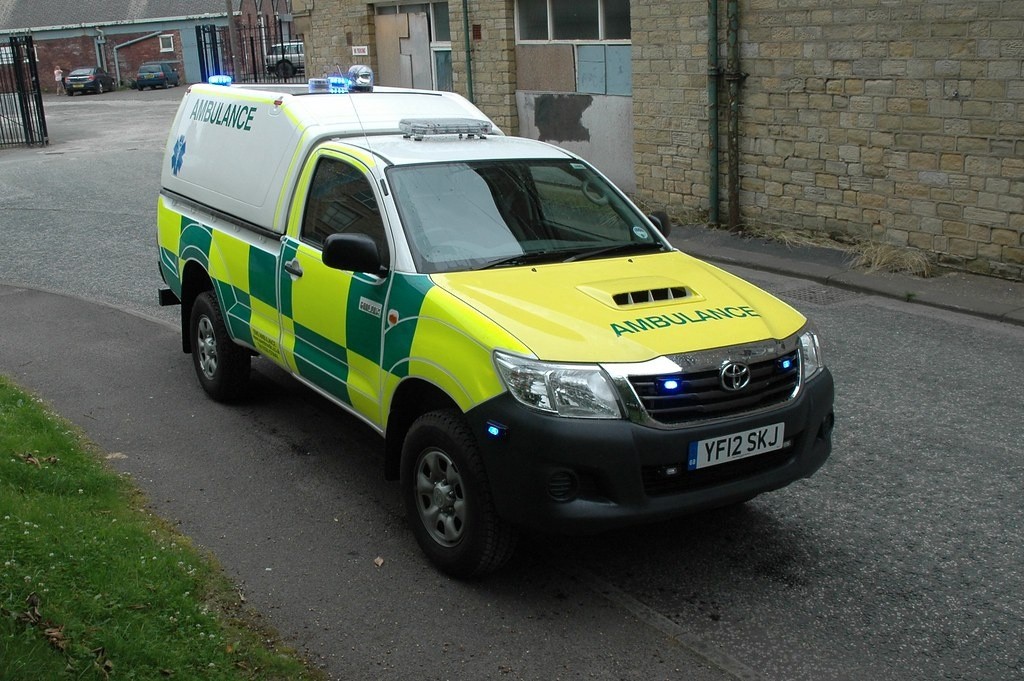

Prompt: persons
[{"left": 54, "top": 66, "right": 67, "bottom": 96}]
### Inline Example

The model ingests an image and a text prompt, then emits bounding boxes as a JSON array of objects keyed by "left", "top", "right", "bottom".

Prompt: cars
[
  {"left": 62, "top": 65, "right": 116, "bottom": 96},
  {"left": 137, "top": 61, "right": 180, "bottom": 91}
]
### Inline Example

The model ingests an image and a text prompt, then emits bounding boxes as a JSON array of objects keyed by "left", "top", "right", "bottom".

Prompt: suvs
[{"left": 266, "top": 41, "right": 305, "bottom": 80}]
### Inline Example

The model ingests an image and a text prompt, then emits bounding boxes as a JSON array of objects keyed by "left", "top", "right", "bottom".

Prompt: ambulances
[{"left": 156, "top": 63, "right": 836, "bottom": 585}]
